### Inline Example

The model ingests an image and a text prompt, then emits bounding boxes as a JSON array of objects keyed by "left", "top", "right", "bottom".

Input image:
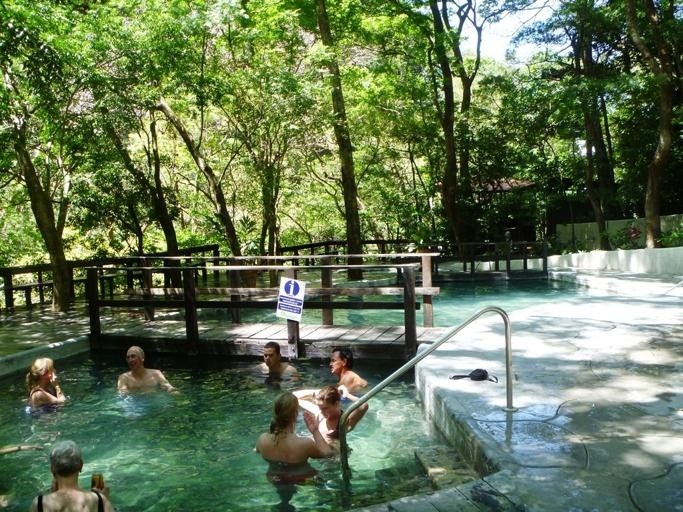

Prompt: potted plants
[
  {"left": 235, "top": 215, "right": 265, "bottom": 288},
  {"left": 413, "top": 225, "right": 437, "bottom": 272}
]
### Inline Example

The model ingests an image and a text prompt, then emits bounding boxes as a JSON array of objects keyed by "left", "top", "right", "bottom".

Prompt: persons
[
  {"left": 26, "top": 439, "right": 115, "bottom": 512},
  {"left": 327, "top": 347, "right": 367, "bottom": 391},
  {"left": 114, "top": 346, "right": 176, "bottom": 395},
  {"left": 25, "top": 355, "right": 69, "bottom": 406},
  {"left": 251, "top": 340, "right": 295, "bottom": 371},
  {"left": 252, "top": 391, "right": 332, "bottom": 462},
  {"left": 287, "top": 384, "right": 368, "bottom": 439}
]
